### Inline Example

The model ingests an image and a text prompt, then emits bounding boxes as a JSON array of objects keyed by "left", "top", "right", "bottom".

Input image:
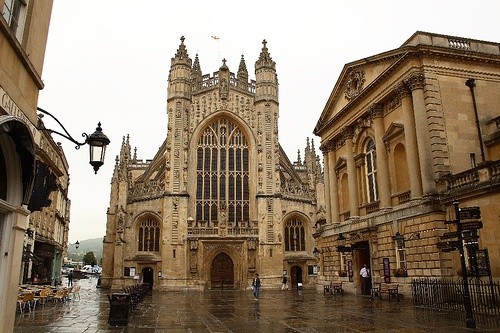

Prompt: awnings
[
  {"left": 24, "top": 246, "right": 44, "bottom": 263},
  {"left": 23, "top": 249, "right": 37, "bottom": 263},
  {"left": 22, "top": 255, "right": 30, "bottom": 262}
]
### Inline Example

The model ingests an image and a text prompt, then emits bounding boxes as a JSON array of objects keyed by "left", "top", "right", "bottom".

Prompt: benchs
[
  {"left": 372, "top": 284, "right": 400, "bottom": 301},
  {"left": 324, "top": 283, "right": 343, "bottom": 296}
]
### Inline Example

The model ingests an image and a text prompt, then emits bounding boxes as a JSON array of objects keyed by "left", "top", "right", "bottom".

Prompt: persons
[
  {"left": 281, "top": 277, "right": 290, "bottom": 291},
  {"left": 252, "top": 274, "right": 260, "bottom": 301},
  {"left": 68, "top": 270, "right": 73, "bottom": 286},
  {"left": 359, "top": 262, "right": 370, "bottom": 295},
  {"left": 220, "top": 78, "right": 227, "bottom": 92}
]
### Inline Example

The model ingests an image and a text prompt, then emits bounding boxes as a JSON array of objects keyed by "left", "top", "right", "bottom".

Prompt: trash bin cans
[
  {"left": 110, "top": 293, "right": 130, "bottom": 326},
  {"left": 55, "top": 277, "right": 61, "bottom": 285}
]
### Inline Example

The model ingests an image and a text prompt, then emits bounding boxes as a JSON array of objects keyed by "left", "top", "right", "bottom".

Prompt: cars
[{"left": 82, "top": 265, "right": 101, "bottom": 275}]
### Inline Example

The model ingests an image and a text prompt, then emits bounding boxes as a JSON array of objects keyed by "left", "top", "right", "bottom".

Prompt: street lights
[{"left": 75, "top": 240, "right": 80, "bottom": 270}]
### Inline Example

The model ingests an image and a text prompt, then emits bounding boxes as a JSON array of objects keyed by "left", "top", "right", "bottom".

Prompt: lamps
[
  {"left": 393, "top": 232, "right": 419, "bottom": 248},
  {"left": 36, "top": 105, "right": 111, "bottom": 174},
  {"left": 68, "top": 240, "right": 80, "bottom": 249},
  {"left": 313, "top": 247, "right": 324, "bottom": 259}
]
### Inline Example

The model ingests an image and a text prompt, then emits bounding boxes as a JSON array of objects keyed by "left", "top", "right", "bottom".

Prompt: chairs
[{"left": 17, "top": 285, "right": 81, "bottom": 313}]
